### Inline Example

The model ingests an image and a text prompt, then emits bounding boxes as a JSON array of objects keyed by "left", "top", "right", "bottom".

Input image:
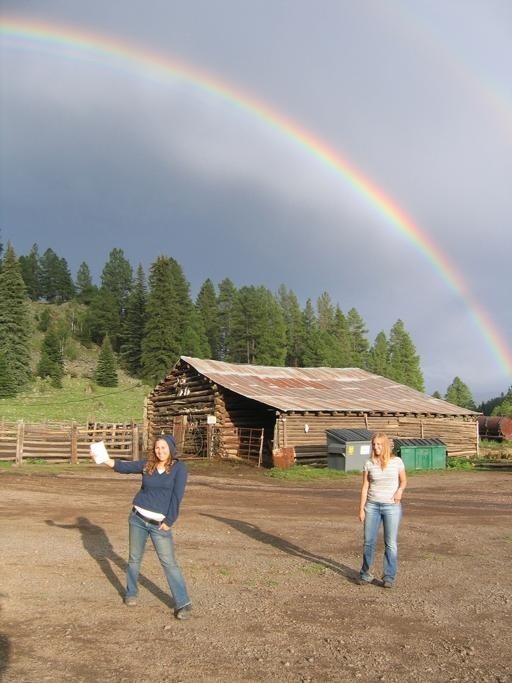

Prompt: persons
[
  {"left": 355, "top": 433, "right": 407, "bottom": 588},
  {"left": 90, "top": 435, "right": 193, "bottom": 620}
]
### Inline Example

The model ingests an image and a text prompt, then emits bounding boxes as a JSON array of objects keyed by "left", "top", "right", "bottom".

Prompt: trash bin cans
[{"left": 393, "top": 438, "right": 447, "bottom": 470}]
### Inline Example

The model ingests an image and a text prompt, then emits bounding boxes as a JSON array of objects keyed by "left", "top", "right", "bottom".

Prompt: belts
[{"left": 132, "top": 507, "right": 164, "bottom": 526}]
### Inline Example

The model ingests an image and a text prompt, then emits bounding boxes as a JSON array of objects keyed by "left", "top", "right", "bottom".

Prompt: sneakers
[
  {"left": 176, "top": 604, "right": 192, "bottom": 620},
  {"left": 355, "top": 579, "right": 369, "bottom": 586},
  {"left": 383, "top": 580, "right": 392, "bottom": 588},
  {"left": 124, "top": 596, "right": 138, "bottom": 607}
]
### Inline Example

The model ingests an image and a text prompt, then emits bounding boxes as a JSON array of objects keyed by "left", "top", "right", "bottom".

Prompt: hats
[{"left": 158, "top": 434, "right": 177, "bottom": 456}]
